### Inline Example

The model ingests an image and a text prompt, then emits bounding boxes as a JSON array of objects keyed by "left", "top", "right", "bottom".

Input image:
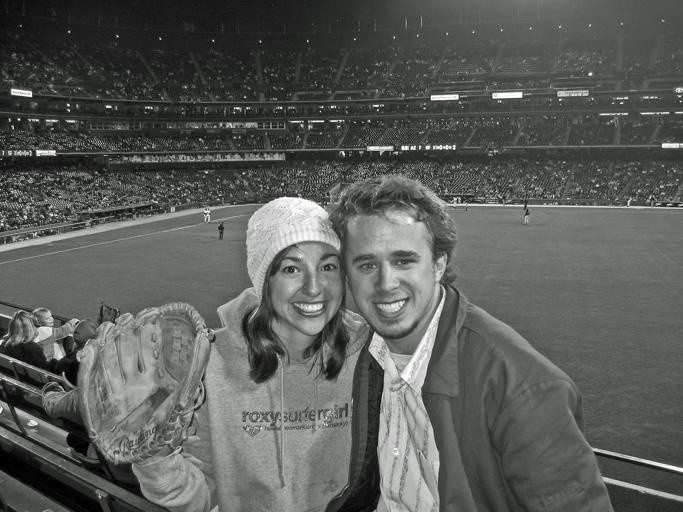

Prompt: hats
[
  {"left": 245, "top": 197, "right": 342, "bottom": 299},
  {"left": 72, "top": 318, "right": 98, "bottom": 344}
]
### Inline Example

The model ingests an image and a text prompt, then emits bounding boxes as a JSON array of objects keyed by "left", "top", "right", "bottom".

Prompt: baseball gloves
[{"left": 77, "top": 302, "right": 214, "bottom": 468}]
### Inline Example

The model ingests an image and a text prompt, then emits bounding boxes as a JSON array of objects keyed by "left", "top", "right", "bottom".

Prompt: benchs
[{"left": 0, "top": 353, "right": 169, "bottom": 512}]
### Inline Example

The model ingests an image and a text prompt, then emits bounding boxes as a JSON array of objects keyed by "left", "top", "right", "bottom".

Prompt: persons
[
  {"left": 73, "top": 194, "right": 374, "bottom": 512},
  {"left": 522, "top": 208, "right": 530, "bottom": 225},
  {"left": 464, "top": 200, "right": 468, "bottom": 212},
  {"left": 314, "top": 170, "right": 615, "bottom": 512},
  {"left": 1, "top": 40, "right": 198, "bottom": 252},
  {"left": 199, "top": 44, "right": 682, "bottom": 208},
  {"left": 0, "top": 305, "right": 104, "bottom": 474},
  {"left": 523, "top": 198, "right": 528, "bottom": 210},
  {"left": 202, "top": 208, "right": 211, "bottom": 225},
  {"left": 216, "top": 221, "right": 224, "bottom": 240}
]
